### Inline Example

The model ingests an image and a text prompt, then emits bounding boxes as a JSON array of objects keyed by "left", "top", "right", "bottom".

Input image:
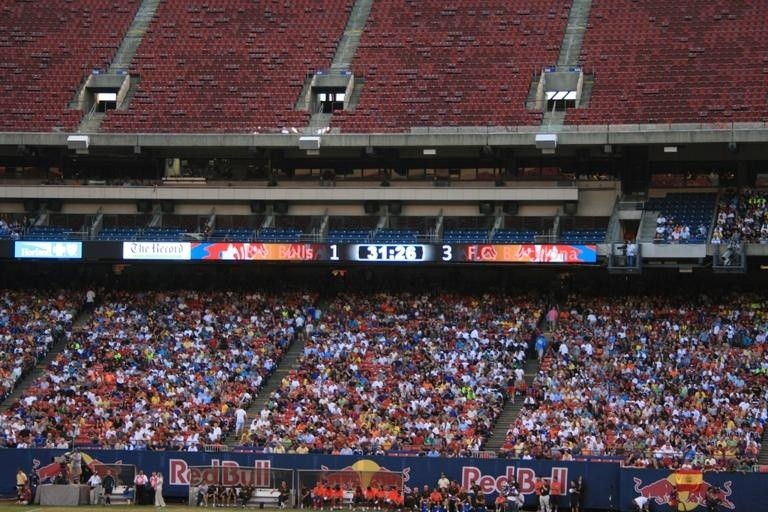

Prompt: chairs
[
  {"left": 407, "top": 501, "right": 412, "bottom": 512},
  {"left": 0, "top": 191, "right": 720, "bottom": 244},
  {"left": 0, "top": 0, "right": 768, "bottom": 133}
]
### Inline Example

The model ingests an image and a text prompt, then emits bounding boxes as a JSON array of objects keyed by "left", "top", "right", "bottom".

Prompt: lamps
[
  {"left": 298, "top": 137, "right": 322, "bottom": 156},
  {"left": 535, "top": 133, "right": 557, "bottom": 155},
  {"left": 67, "top": 136, "right": 90, "bottom": 156}
]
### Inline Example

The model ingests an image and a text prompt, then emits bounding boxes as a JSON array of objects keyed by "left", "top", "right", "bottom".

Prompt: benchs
[
  {"left": 310, "top": 490, "right": 356, "bottom": 512},
  {"left": 189, "top": 487, "right": 296, "bottom": 509},
  {"left": 99, "top": 494, "right": 133, "bottom": 505}
]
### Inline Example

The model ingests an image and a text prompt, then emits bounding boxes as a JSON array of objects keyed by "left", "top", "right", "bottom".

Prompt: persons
[{"left": 0, "top": 191, "right": 767, "bottom": 512}]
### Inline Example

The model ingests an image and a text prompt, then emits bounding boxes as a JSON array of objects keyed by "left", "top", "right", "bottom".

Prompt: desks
[{"left": 33, "top": 484, "right": 91, "bottom": 506}]
